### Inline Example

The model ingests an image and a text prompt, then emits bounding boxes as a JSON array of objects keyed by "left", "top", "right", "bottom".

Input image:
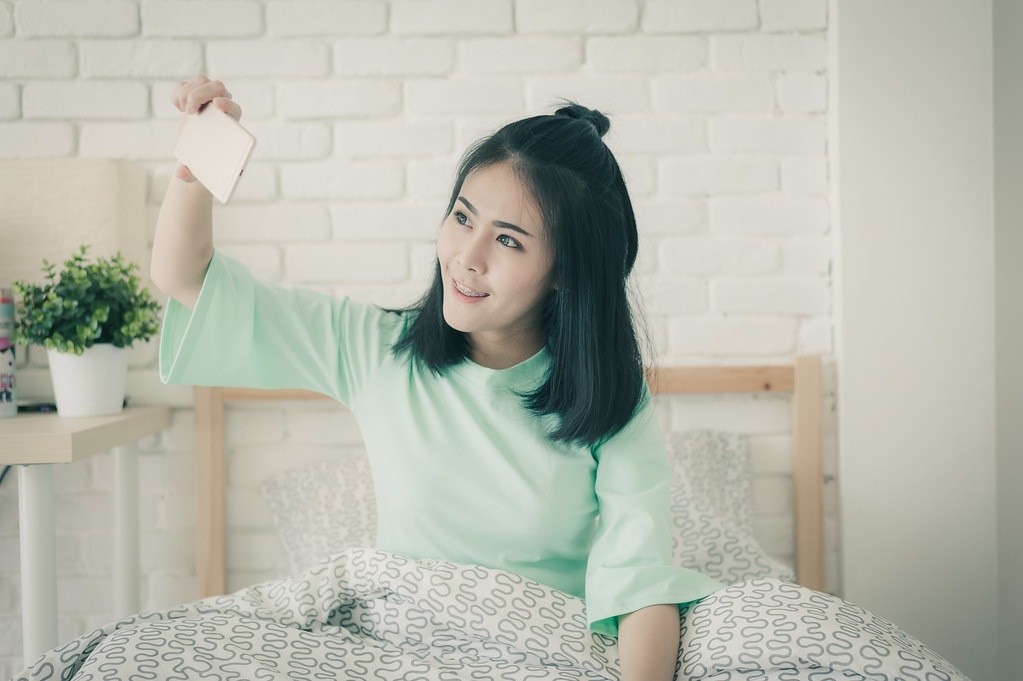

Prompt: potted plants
[{"left": 11, "top": 245, "right": 161, "bottom": 417}]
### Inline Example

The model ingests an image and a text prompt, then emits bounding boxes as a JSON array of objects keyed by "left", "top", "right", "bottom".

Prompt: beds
[{"left": 6, "top": 353, "right": 826, "bottom": 681}]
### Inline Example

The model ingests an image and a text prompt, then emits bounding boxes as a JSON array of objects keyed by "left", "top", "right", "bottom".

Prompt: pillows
[{"left": 268, "top": 430, "right": 794, "bottom": 591}]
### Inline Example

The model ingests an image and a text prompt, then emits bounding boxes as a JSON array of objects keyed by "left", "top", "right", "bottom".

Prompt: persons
[{"left": 150, "top": 74, "right": 728, "bottom": 681}]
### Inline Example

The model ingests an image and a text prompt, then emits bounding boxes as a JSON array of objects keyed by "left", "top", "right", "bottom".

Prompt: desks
[{"left": 0, "top": 408, "right": 173, "bottom": 664}]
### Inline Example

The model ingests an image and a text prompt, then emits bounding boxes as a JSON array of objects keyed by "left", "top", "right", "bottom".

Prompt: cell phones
[{"left": 176, "top": 101, "right": 255, "bottom": 204}]
